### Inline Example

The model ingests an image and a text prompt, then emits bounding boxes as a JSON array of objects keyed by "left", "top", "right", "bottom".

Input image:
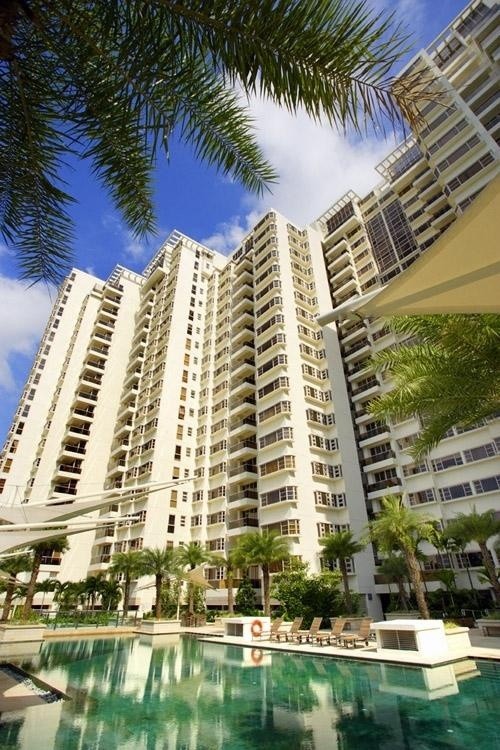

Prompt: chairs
[
  {"left": 270, "top": 617, "right": 303, "bottom": 643},
  {"left": 292, "top": 617, "right": 323, "bottom": 644},
  {"left": 252, "top": 618, "right": 283, "bottom": 641},
  {"left": 290, "top": 652, "right": 377, "bottom": 679},
  {"left": 341, "top": 618, "right": 373, "bottom": 648},
  {"left": 312, "top": 617, "right": 347, "bottom": 646}
]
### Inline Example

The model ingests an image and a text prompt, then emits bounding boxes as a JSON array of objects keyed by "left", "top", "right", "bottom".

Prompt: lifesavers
[
  {"left": 251, "top": 620, "right": 263, "bottom": 636},
  {"left": 252, "top": 648, "right": 262, "bottom": 663}
]
